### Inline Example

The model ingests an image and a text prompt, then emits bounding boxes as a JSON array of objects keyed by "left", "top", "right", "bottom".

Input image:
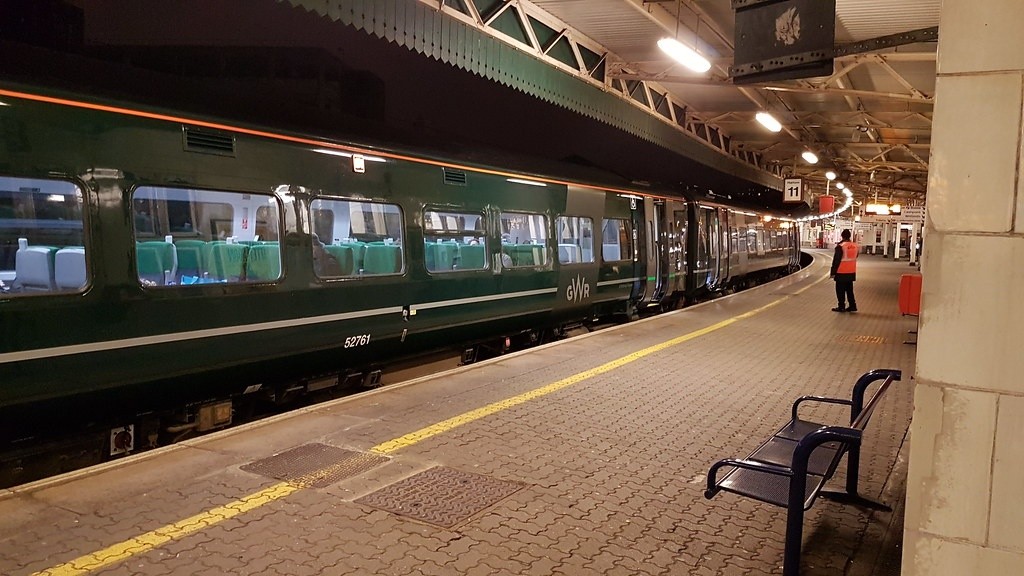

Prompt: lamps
[
  {"left": 754, "top": 110, "right": 783, "bottom": 132},
  {"left": 657, "top": 37, "right": 712, "bottom": 73}
]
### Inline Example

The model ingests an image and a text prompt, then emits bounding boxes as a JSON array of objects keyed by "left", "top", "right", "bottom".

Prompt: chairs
[
  {"left": 325, "top": 239, "right": 403, "bottom": 276},
  {"left": 16, "top": 234, "right": 85, "bottom": 290},
  {"left": 503, "top": 242, "right": 544, "bottom": 266},
  {"left": 559, "top": 244, "right": 591, "bottom": 263},
  {"left": 423, "top": 237, "right": 485, "bottom": 271},
  {"left": 135, "top": 235, "right": 280, "bottom": 285},
  {"left": 603, "top": 244, "right": 622, "bottom": 261}
]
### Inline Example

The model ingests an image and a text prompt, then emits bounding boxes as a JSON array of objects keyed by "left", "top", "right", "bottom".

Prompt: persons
[
  {"left": 502, "top": 246, "right": 513, "bottom": 268},
  {"left": 312, "top": 232, "right": 342, "bottom": 276},
  {"left": 829, "top": 229, "right": 859, "bottom": 312},
  {"left": 915, "top": 237, "right": 921, "bottom": 271}
]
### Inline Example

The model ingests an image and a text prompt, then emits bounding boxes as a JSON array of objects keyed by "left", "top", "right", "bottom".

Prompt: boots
[
  {"left": 846, "top": 303, "right": 857, "bottom": 312},
  {"left": 831, "top": 303, "right": 846, "bottom": 312}
]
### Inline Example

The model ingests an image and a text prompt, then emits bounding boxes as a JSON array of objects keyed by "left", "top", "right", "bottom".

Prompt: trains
[{"left": 1, "top": 81, "right": 800, "bottom": 447}]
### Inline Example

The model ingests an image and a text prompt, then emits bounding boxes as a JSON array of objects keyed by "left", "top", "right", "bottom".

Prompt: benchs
[{"left": 703, "top": 369, "right": 902, "bottom": 576}]
